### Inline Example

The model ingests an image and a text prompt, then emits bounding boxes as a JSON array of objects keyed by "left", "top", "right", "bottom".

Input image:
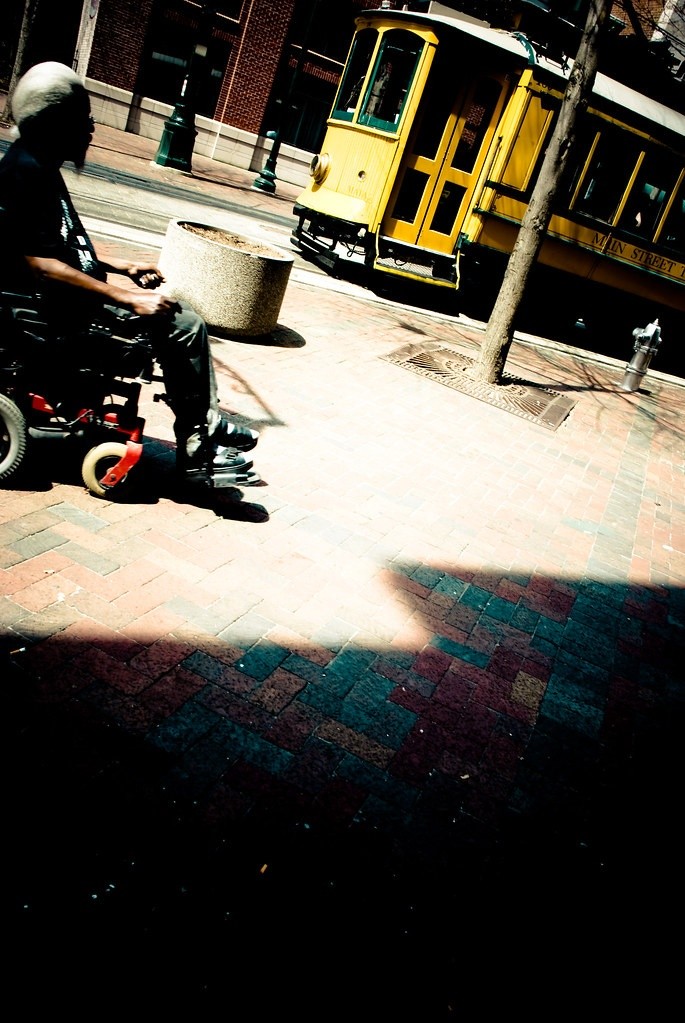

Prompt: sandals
[
  {"left": 178, "top": 439, "right": 253, "bottom": 475},
  {"left": 209, "top": 418, "right": 257, "bottom": 451}
]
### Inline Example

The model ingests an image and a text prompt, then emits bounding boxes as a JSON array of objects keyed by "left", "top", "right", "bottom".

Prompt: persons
[{"left": 0, "top": 62, "right": 260, "bottom": 485}]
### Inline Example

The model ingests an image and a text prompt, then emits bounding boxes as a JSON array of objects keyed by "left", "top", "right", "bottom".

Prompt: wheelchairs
[{"left": 0, "top": 280, "right": 159, "bottom": 502}]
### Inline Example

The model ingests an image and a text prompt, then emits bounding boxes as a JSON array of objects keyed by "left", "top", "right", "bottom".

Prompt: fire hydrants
[{"left": 619, "top": 319, "right": 663, "bottom": 393}]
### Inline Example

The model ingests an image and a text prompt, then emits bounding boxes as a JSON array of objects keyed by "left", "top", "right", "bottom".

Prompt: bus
[{"left": 289, "top": 0, "right": 685, "bottom": 340}]
